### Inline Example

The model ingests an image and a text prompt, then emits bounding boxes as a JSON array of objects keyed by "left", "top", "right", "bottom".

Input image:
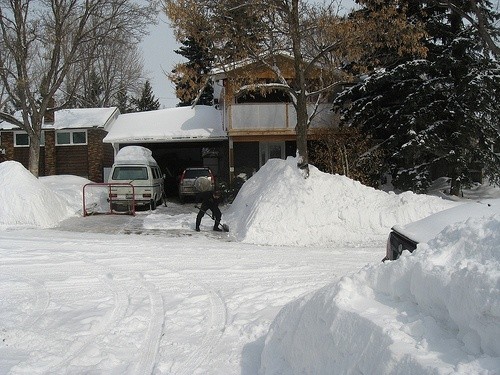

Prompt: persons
[{"left": 195, "top": 191, "right": 223, "bottom": 232}]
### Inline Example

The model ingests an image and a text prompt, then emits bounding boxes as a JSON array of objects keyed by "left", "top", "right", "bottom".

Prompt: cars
[{"left": 381, "top": 198, "right": 500, "bottom": 264}]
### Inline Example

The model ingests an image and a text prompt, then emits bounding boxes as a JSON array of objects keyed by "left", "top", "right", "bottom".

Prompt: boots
[
  {"left": 213, "top": 220, "right": 222, "bottom": 231},
  {"left": 196, "top": 218, "right": 201, "bottom": 231}
]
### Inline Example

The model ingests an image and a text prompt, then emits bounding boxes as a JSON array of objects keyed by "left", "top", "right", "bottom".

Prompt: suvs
[
  {"left": 179, "top": 168, "right": 216, "bottom": 200},
  {"left": 107, "top": 160, "right": 167, "bottom": 211}
]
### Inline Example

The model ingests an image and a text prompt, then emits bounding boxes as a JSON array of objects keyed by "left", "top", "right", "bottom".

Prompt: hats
[{"left": 213, "top": 188, "right": 221, "bottom": 196}]
